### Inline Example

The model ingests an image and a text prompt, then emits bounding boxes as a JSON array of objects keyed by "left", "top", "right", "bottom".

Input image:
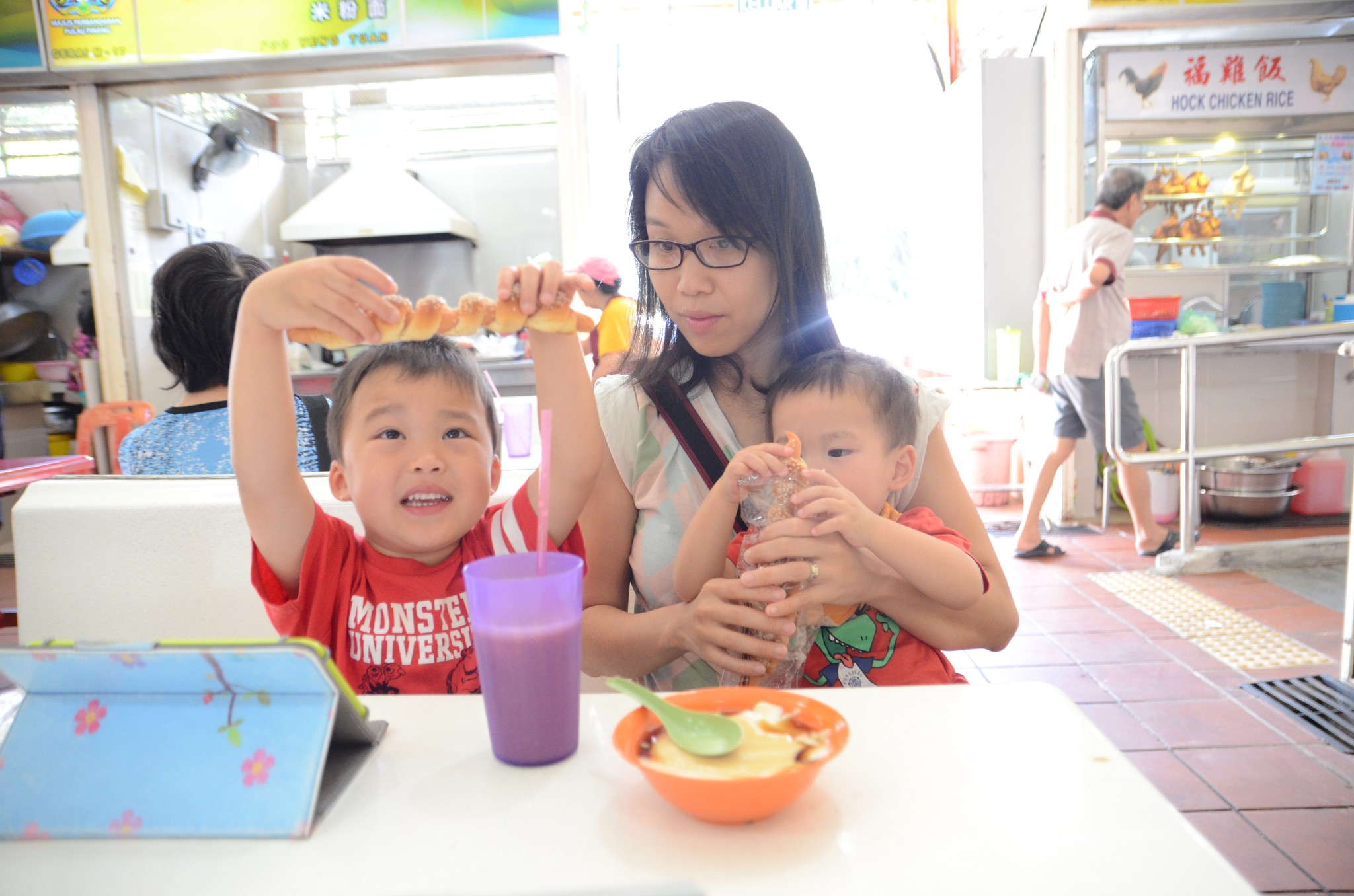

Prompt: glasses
[{"left": 629, "top": 229, "right": 766, "bottom": 270}]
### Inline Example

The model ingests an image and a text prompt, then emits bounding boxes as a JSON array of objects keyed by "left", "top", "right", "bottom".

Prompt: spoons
[{"left": 607, "top": 676, "right": 742, "bottom": 756}]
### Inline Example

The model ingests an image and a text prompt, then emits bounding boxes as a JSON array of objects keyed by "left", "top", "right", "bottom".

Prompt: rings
[{"left": 804, "top": 560, "right": 820, "bottom": 586}]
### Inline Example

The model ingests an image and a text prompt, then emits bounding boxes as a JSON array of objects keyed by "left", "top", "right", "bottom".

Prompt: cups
[{"left": 464, "top": 551, "right": 583, "bottom": 765}]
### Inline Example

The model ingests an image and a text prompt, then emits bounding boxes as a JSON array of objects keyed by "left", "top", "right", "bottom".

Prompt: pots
[{"left": 41, "top": 392, "right": 78, "bottom": 436}]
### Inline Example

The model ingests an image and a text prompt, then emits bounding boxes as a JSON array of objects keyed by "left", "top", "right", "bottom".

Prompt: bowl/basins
[
  {"left": 610, "top": 687, "right": 858, "bottom": 820},
  {"left": 1196, "top": 454, "right": 1303, "bottom": 493},
  {"left": 1197, "top": 482, "right": 1304, "bottom": 518},
  {"left": 33, "top": 360, "right": 73, "bottom": 382},
  {"left": 0, "top": 362, "right": 37, "bottom": 383}
]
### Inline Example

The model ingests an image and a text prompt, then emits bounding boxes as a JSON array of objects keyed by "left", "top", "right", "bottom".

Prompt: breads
[{"left": 285, "top": 293, "right": 600, "bottom": 350}]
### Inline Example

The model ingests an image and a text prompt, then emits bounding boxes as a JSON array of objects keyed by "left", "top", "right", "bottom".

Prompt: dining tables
[{"left": 3, "top": 680, "right": 1259, "bottom": 896}]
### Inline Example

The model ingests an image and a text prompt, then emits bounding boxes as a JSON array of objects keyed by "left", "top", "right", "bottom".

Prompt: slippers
[
  {"left": 1015, "top": 539, "right": 1066, "bottom": 558},
  {"left": 1140, "top": 529, "right": 1200, "bottom": 556}
]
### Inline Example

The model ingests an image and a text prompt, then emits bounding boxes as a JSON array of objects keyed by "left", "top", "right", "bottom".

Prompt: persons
[
  {"left": 227, "top": 253, "right": 604, "bottom": 697},
  {"left": 571, "top": 258, "right": 665, "bottom": 383},
  {"left": 115, "top": 237, "right": 319, "bottom": 477},
  {"left": 568, "top": 100, "right": 1021, "bottom": 687},
  {"left": 1013, "top": 163, "right": 1200, "bottom": 559},
  {"left": 680, "top": 352, "right": 991, "bottom": 687}
]
